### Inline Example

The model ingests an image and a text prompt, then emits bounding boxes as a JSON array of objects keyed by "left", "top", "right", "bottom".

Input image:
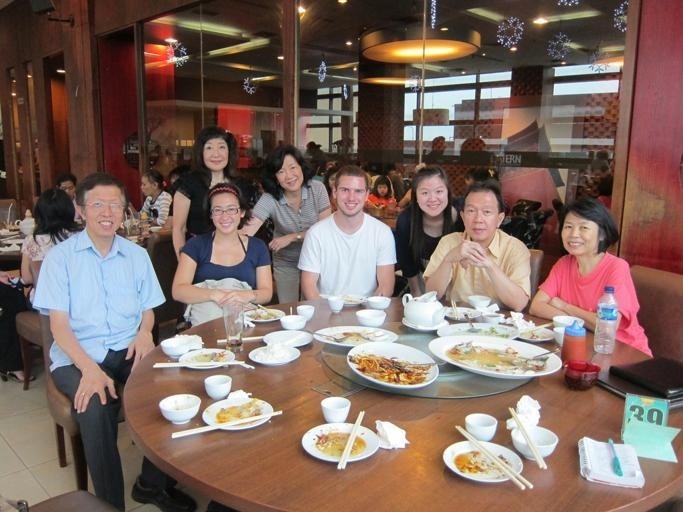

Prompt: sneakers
[{"left": 131, "top": 474, "right": 197, "bottom": 512}]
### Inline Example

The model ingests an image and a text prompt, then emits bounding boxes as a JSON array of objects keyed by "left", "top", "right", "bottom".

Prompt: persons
[
  {"left": 528, "top": 196, "right": 653, "bottom": 358},
  {"left": 139, "top": 123, "right": 491, "bottom": 332},
  {"left": 20, "top": 187, "right": 82, "bottom": 311},
  {"left": 56, "top": 173, "right": 85, "bottom": 222},
  {"left": 425, "top": 179, "right": 531, "bottom": 314},
  {"left": 0, "top": 280, "right": 37, "bottom": 384},
  {"left": 32, "top": 171, "right": 199, "bottom": 511}
]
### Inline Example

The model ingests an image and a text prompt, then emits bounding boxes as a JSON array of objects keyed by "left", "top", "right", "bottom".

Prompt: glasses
[
  {"left": 210, "top": 207, "right": 242, "bottom": 216},
  {"left": 77, "top": 200, "right": 126, "bottom": 213}
]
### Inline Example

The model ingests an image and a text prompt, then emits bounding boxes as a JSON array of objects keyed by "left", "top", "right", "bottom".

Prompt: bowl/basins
[
  {"left": 366, "top": 296, "right": 391, "bottom": 309},
  {"left": 356, "top": 308, "right": 387, "bottom": 327},
  {"left": 160, "top": 336, "right": 202, "bottom": 359},
  {"left": 280, "top": 315, "right": 306, "bottom": 329},
  {"left": 510, "top": 426, "right": 560, "bottom": 461},
  {"left": 553, "top": 327, "right": 565, "bottom": 348},
  {"left": 552, "top": 315, "right": 585, "bottom": 328},
  {"left": 483, "top": 313, "right": 505, "bottom": 324},
  {"left": 158, "top": 393, "right": 202, "bottom": 425},
  {"left": 469, "top": 295, "right": 491, "bottom": 308}
]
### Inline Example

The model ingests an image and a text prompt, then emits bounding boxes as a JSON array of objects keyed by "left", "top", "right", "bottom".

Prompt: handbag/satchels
[{"left": 183, "top": 277, "right": 256, "bottom": 329}]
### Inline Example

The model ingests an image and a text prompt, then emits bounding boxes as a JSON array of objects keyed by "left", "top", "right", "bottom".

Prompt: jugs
[{"left": 401, "top": 291, "right": 448, "bottom": 327}]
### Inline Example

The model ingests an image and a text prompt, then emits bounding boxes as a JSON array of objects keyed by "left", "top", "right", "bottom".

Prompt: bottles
[
  {"left": 561, "top": 320, "right": 586, "bottom": 359},
  {"left": 594, "top": 286, "right": 619, "bottom": 356}
]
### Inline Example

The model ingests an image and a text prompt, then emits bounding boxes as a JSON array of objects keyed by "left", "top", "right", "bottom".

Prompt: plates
[
  {"left": 248, "top": 346, "right": 301, "bottom": 366},
  {"left": 429, "top": 334, "right": 563, "bottom": 380},
  {"left": 332, "top": 294, "right": 366, "bottom": 307},
  {"left": 302, "top": 422, "right": 381, "bottom": 463},
  {"left": 262, "top": 330, "right": 313, "bottom": 348},
  {"left": 443, "top": 441, "right": 523, "bottom": 482},
  {"left": 240, "top": 309, "right": 285, "bottom": 323},
  {"left": 203, "top": 397, "right": 273, "bottom": 431},
  {"left": 518, "top": 325, "right": 554, "bottom": 344},
  {"left": 119, "top": 212, "right": 164, "bottom": 242},
  {"left": 314, "top": 326, "right": 398, "bottom": 348},
  {"left": 446, "top": 306, "right": 482, "bottom": 321},
  {"left": 436, "top": 322, "right": 520, "bottom": 340},
  {"left": 178, "top": 348, "right": 235, "bottom": 370},
  {"left": 401, "top": 318, "right": 450, "bottom": 332},
  {"left": 346, "top": 341, "right": 440, "bottom": 390},
  {"left": 1, "top": 210, "right": 37, "bottom": 252}
]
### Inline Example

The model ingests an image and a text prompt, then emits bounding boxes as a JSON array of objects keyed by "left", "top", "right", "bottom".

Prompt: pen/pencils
[{"left": 609, "top": 438, "right": 623, "bottom": 476}]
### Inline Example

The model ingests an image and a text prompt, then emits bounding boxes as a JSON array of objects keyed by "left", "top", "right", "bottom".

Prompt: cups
[
  {"left": 329, "top": 297, "right": 344, "bottom": 313},
  {"left": 465, "top": 411, "right": 498, "bottom": 440},
  {"left": 370, "top": 207, "right": 385, "bottom": 217},
  {"left": 205, "top": 374, "right": 233, "bottom": 401},
  {"left": 563, "top": 359, "right": 600, "bottom": 391},
  {"left": 297, "top": 305, "right": 314, "bottom": 321},
  {"left": 224, "top": 300, "right": 243, "bottom": 351},
  {"left": 319, "top": 397, "right": 351, "bottom": 423}
]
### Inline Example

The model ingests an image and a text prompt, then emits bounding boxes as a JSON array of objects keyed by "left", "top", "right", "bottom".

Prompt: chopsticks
[
  {"left": 454, "top": 424, "right": 535, "bottom": 490},
  {"left": 154, "top": 361, "right": 245, "bottom": 368},
  {"left": 337, "top": 410, "right": 364, "bottom": 470},
  {"left": 172, "top": 409, "right": 282, "bottom": 439},
  {"left": 510, "top": 406, "right": 547, "bottom": 469},
  {"left": 216, "top": 335, "right": 262, "bottom": 342}
]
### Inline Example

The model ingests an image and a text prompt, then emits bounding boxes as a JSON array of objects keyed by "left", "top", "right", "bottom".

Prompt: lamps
[{"left": 359, "top": 0, "right": 482, "bottom": 86}]
